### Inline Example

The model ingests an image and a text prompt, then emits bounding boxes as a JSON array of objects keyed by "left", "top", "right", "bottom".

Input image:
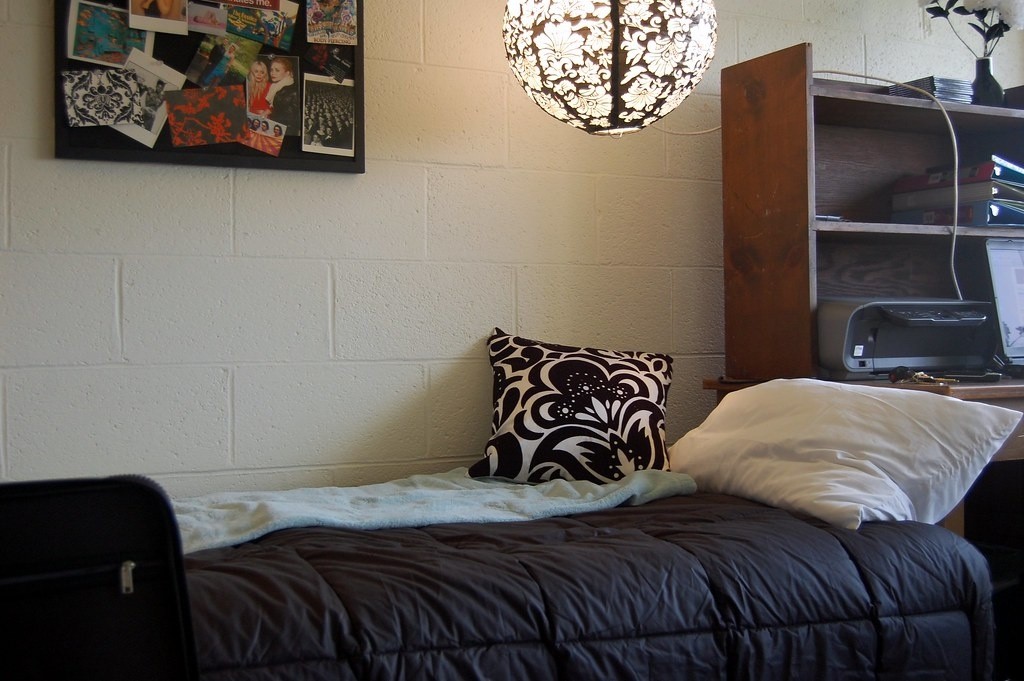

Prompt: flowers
[{"left": 919, "top": 0, "right": 1024, "bottom": 59}]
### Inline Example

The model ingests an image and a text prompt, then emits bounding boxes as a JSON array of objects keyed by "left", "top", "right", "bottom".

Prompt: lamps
[{"left": 502, "top": 0, "right": 718, "bottom": 139}]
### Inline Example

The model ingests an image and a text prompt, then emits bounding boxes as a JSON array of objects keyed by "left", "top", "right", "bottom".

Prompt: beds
[{"left": 0, "top": 470, "right": 996, "bottom": 681}]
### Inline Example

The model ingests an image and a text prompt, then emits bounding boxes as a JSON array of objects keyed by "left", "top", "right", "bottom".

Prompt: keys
[{"left": 923, "top": 377, "right": 960, "bottom": 383}]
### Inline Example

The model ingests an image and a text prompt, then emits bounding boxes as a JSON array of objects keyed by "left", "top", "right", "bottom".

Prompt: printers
[{"left": 814, "top": 294, "right": 999, "bottom": 381}]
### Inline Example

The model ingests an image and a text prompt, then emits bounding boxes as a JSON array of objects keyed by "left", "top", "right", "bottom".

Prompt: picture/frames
[{"left": 54, "top": 0, "right": 365, "bottom": 173}]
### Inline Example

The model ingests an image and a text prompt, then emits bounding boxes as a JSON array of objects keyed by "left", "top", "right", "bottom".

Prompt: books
[{"left": 889, "top": 76, "right": 973, "bottom": 104}]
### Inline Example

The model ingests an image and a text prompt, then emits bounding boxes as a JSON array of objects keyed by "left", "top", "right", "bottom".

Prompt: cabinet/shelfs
[{"left": 700, "top": 41, "right": 1024, "bottom": 539}]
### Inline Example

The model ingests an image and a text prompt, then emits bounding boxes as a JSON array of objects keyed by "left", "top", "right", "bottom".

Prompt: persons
[
  {"left": 146, "top": 80, "right": 165, "bottom": 112},
  {"left": 194, "top": 11, "right": 219, "bottom": 25},
  {"left": 248, "top": 58, "right": 299, "bottom": 139},
  {"left": 197, "top": 38, "right": 236, "bottom": 87},
  {"left": 306, "top": 91, "right": 354, "bottom": 148}
]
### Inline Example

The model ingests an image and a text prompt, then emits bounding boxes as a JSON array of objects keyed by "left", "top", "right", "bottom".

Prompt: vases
[{"left": 971, "top": 57, "right": 1004, "bottom": 107}]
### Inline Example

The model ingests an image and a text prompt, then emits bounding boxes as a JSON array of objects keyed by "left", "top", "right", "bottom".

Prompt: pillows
[
  {"left": 465, "top": 326, "right": 675, "bottom": 485},
  {"left": 667, "top": 378, "right": 1024, "bottom": 529}
]
